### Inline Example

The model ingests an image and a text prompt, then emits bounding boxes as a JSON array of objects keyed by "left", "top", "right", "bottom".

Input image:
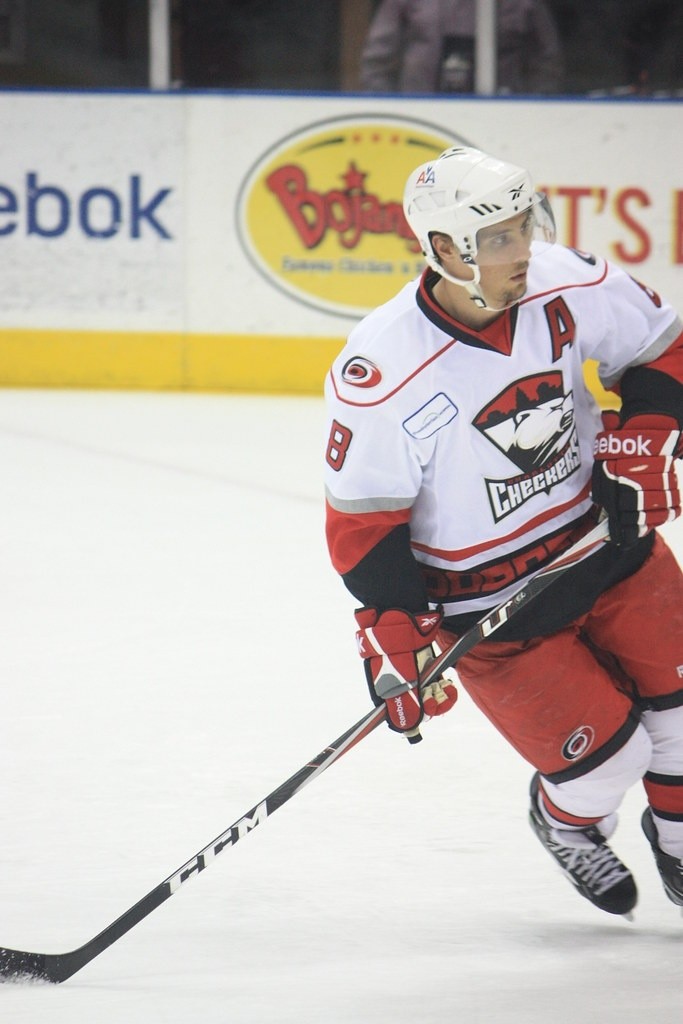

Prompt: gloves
[
  {"left": 354, "top": 603, "right": 459, "bottom": 733},
  {"left": 589, "top": 410, "right": 683, "bottom": 559}
]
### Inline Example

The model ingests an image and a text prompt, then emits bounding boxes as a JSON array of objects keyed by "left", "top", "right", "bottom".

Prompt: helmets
[{"left": 402, "top": 145, "right": 542, "bottom": 287}]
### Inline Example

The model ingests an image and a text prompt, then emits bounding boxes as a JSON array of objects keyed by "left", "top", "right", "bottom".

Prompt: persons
[
  {"left": 319, "top": 147, "right": 683, "bottom": 919},
  {"left": 358, "top": 0, "right": 565, "bottom": 96}
]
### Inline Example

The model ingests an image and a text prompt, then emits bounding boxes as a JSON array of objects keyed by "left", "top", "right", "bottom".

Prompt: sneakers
[
  {"left": 640, "top": 805, "right": 683, "bottom": 907},
  {"left": 526, "top": 771, "right": 638, "bottom": 915}
]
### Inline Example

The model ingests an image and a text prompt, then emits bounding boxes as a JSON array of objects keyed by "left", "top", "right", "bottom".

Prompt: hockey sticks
[{"left": 0, "top": 514, "right": 609, "bottom": 984}]
